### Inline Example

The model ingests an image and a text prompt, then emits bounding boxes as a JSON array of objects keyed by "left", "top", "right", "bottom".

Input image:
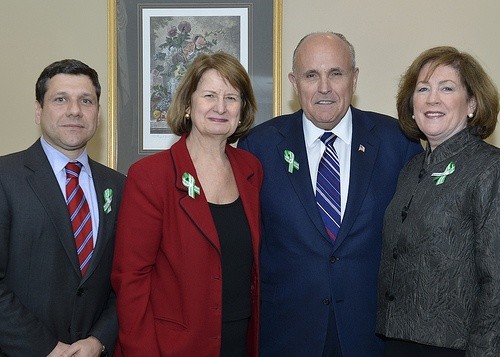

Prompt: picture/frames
[{"left": 106, "top": 0, "right": 284, "bottom": 177}]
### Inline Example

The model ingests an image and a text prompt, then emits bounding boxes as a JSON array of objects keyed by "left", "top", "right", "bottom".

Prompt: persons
[
  {"left": 0, "top": 59, "right": 125, "bottom": 357},
  {"left": 374, "top": 47, "right": 500, "bottom": 357},
  {"left": 112, "top": 53, "right": 265, "bottom": 357},
  {"left": 237, "top": 33, "right": 426, "bottom": 357}
]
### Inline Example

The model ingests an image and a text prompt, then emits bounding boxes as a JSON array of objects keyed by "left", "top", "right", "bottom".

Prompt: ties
[
  {"left": 314, "top": 132, "right": 341, "bottom": 245},
  {"left": 64, "top": 163, "right": 94, "bottom": 277}
]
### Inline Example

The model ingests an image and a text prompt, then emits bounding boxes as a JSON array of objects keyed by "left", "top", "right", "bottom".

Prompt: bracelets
[{"left": 102, "top": 346, "right": 105, "bottom": 352}]
